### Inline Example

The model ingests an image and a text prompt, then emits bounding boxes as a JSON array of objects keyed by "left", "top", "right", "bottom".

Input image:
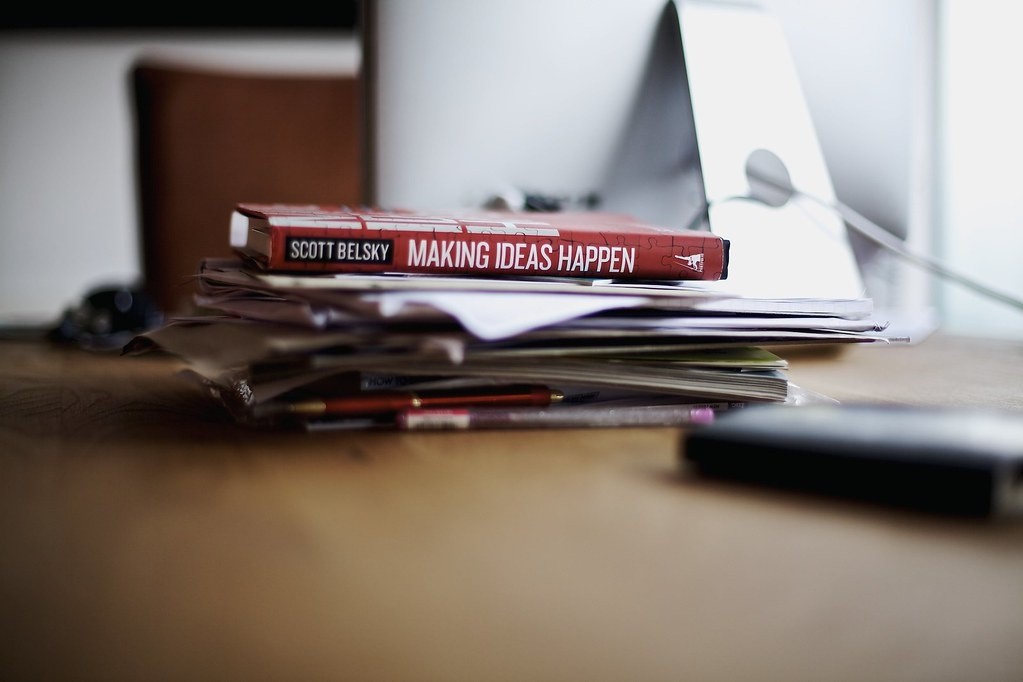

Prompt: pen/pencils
[{"left": 250, "top": 393, "right": 713, "bottom": 430}]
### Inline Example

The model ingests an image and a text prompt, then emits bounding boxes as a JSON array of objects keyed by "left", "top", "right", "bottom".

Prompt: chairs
[{"left": 126, "top": 57, "right": 365, "bottom": 312}]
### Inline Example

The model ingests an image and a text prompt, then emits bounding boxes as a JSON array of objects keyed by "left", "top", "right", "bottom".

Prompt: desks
[{"left": 0, "top": 323, "right": 1023, "bottom": 682}]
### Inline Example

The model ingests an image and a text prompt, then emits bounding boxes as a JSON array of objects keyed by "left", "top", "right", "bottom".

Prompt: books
[
  {"left": 678, "top": 400, "right": 1023, "bottom": 521},
  {"left": 118, "top": 202, "right": 891, "bottom": 433}
]
